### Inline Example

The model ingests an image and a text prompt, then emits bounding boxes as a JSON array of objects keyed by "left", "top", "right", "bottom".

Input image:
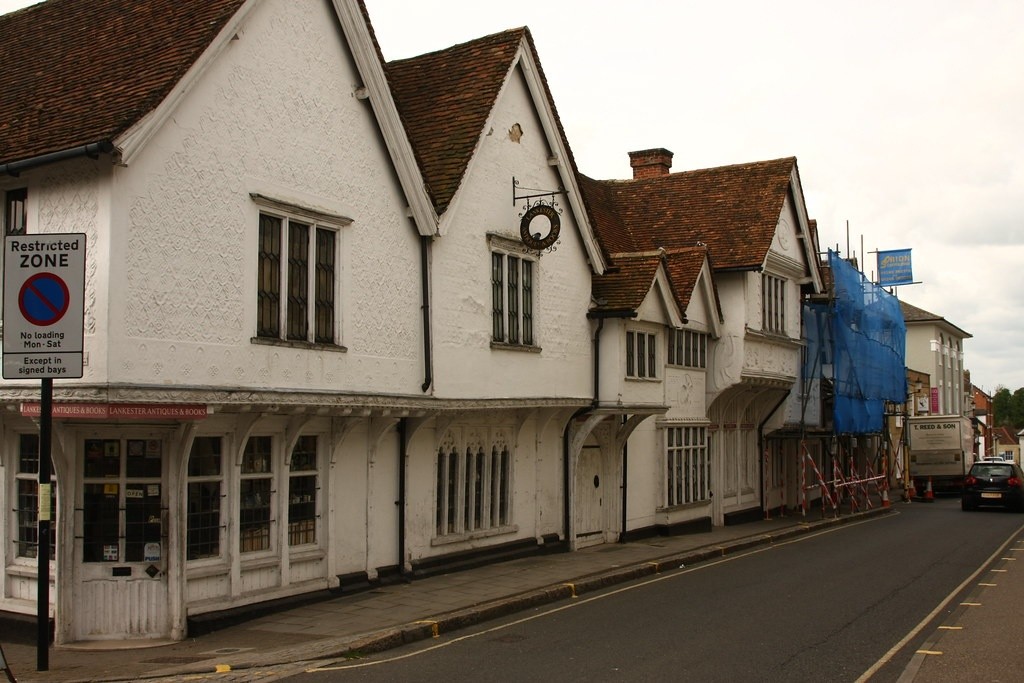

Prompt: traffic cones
[
  {"left": 922, "top": 476, "right": 935, "bottom": 503},
  {"left": 909, "top": 477, "right": 916, "bottom": 497},
  {"left": 881, "top": 488, "right": 889, "bottom": 505}
]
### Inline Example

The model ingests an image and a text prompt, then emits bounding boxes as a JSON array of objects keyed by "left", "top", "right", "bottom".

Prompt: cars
[{"left": 961, "top": 456, "right": 1024, "bottom": 512}]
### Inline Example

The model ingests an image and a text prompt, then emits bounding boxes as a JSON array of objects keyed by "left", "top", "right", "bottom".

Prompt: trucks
[{"left": 907, "top": 414, "right": 978, "bottom": 496}]
